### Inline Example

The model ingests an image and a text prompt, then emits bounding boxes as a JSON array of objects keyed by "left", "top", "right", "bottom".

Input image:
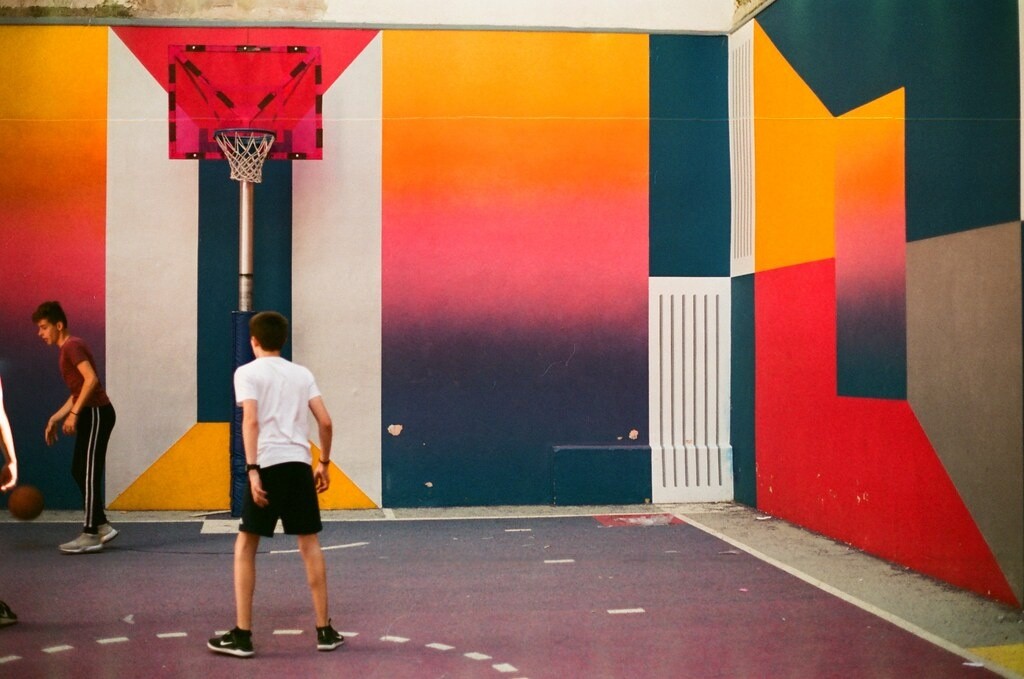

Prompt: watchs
[{"left": 244, "top": 463, "right": 260, "bottom": 473}]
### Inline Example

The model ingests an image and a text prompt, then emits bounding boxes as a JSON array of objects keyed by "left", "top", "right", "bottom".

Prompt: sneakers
[
  {"left": 96, "top": 525, "right": 119, "bottom": 542},
  {"left": 0, "top": 601, "right": 19, "bottom": 626},
  {"left": 316, "top": 621, "right": 345, "bottom": 650},
  {"left": 58, "top": 533, "right": 103, "bottom": 553},
  {"left": 207, "top": 630, "right": 255, "bottom": 658}
]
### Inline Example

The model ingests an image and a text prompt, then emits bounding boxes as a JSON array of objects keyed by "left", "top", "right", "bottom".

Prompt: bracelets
[
  {"left": 70, "top": 410, "right": 79, "bottom": 415},
  {"left": 319, "top": 457, "right": 331, "bottom": 464}
]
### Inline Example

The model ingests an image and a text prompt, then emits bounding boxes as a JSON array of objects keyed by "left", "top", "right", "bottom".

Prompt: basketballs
[{"left": 9, "top": 485, "right": 43, "bottom": 520}]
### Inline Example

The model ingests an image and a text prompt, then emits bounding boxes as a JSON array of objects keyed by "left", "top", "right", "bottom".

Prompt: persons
[
  {"left": 30, "top": 298, "right": 119, "bottom": 555},
  {"left": 205, "top": 310, "right": 346, "bottom": 658},
  {"left": 0, "top": 376, "right": 19, "bottom": 626}
]
